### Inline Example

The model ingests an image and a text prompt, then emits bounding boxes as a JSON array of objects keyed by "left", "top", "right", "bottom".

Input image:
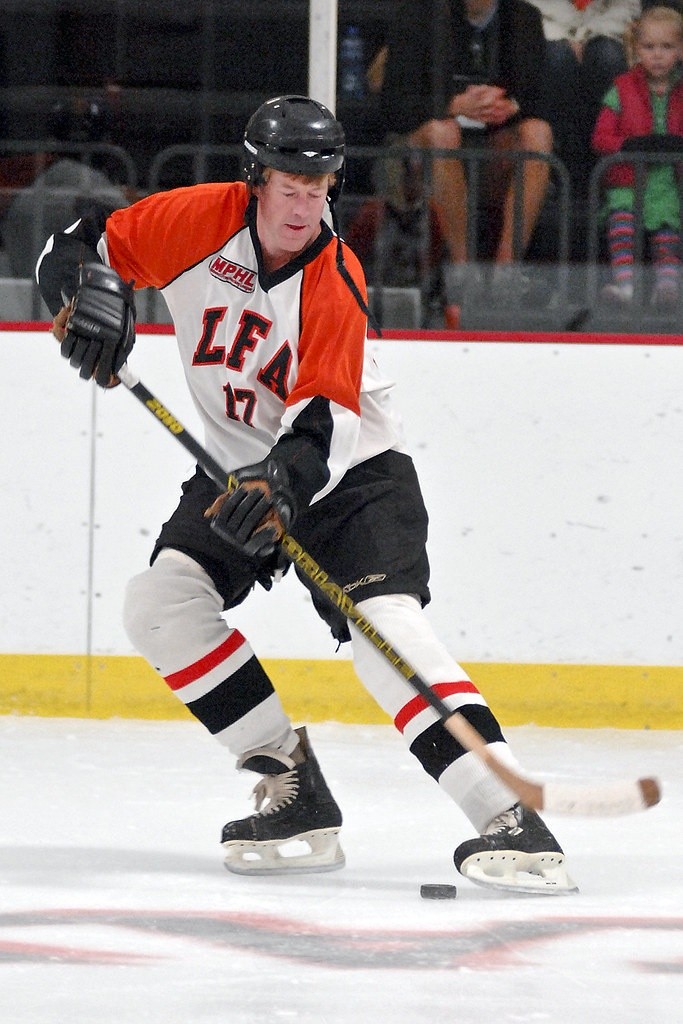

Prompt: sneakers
[
  {"left": 220, "top": 726, "right": 346, "bottom": 876},
  {"left": 453, "top": 799, "right": 581, "bottom": 896}
]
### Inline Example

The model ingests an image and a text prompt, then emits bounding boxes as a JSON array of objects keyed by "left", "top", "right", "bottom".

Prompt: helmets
[{"left": 245, "top": 94, "right": 346, "bottom": 205}]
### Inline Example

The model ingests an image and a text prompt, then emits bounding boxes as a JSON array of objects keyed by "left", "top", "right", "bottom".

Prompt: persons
[
  {"left": 36, "top": 96, "right": 578, "bottom": 894},
  {"left": 380, "top": 0, "right": 683, "bottom": 309}
]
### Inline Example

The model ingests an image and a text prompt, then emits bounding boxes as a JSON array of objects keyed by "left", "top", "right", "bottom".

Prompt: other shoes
[
  {"left": 604, "top": 279, "right": 637, "bottom": 307},
  {"left": 650, "top": 278, "right": 680, "bottom": 309}
]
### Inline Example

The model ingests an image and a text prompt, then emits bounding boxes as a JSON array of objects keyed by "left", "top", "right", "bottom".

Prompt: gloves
[
  {"left": 52, "top": 261, "right": 137, "bottom": 389},
  {"left": 204, "top": 433, "right": 332, "bottom": 562}
]
